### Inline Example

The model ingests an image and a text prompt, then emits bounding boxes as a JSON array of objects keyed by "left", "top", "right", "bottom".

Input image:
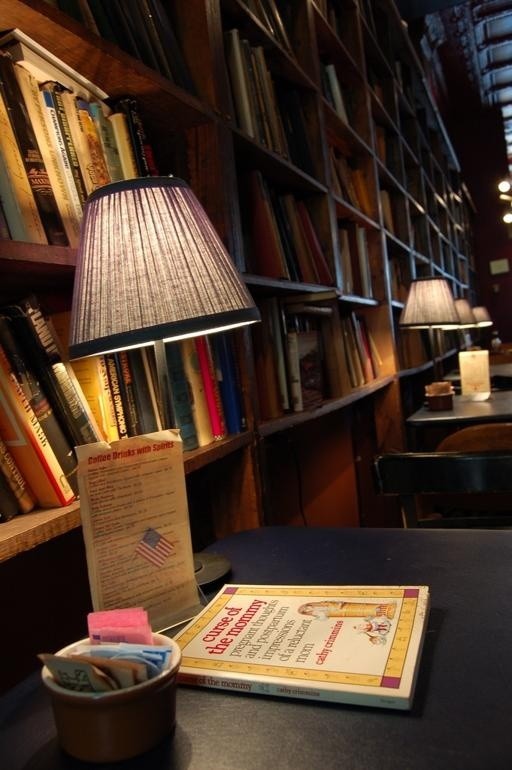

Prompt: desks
[
  {"left": 5, "top": 521, "right": 510, "bottom": 764},
  {"left": 404, "top": 363, "right": 512, "bottom": 426}
]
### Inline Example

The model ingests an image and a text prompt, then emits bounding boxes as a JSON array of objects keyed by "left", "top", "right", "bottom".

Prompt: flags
[{"left": 130, "top": 526, "right": 175, "bottom": 570}]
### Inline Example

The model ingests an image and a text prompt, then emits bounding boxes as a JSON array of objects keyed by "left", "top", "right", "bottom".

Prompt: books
[
  {"left": 1, "top": 2, "right": 247, "bottom": 523},
  {"left": 172, "top": 584, "right": 432, "bottom": 711},
  {"left": 220, "top": 1, "right": 334, "bottom": 426},
  {"left": 310, "top": 2, "right": 404, "bottom": 389}
]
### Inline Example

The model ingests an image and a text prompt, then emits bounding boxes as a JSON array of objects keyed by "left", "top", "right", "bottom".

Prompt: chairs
[{"left": 366, "top": 422, "right": 512, "bottom": 529}]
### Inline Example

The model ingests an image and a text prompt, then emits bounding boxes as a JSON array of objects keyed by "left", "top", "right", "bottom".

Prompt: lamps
[
  {"left": 398, "top": 273, "right": 494, "bottom": 334},
  {"left": 67, "top": 177, "right": 266, "bottom": 598}
]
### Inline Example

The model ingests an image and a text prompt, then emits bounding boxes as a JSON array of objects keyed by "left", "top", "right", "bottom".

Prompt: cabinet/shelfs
[{"left": 2, "top": 0, "right": 479, "bottom": 560}]
